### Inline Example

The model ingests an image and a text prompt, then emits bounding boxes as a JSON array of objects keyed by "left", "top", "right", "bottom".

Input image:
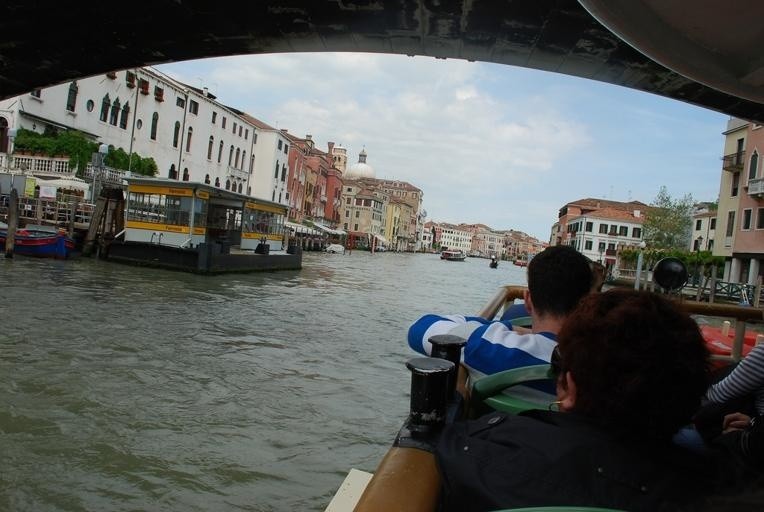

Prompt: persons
[
  {"left": 701, "top": 324, "right": 764, "bottom": 402},
  {"left": 402, "top": 244, "right": 596, "bottom": 441},
  {"left": 429, "top": 285, "right": 711, "bottom": 512},
  {"left": 717, "top": 406, "right": 764, "bottom": 438}
]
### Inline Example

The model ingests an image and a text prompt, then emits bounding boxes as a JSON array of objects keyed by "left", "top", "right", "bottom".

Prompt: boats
[
  {"left": 513, "top": 254, "right": 528, "bottom": 266},
  {"left": 0, "top": 221, "right": 75, "bottom": 260},
  {"left": 439, "top": 246, "right": 466, "bottom": 262},
  {"left": 490, "top": 259, "right": 497, "bottom": 268}
]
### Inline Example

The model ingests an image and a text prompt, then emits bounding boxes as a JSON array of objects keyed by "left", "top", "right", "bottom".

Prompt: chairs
[
  {"left": 471, "top": 363, "right": 558, "bottom": 416},
  {"left": 508, "top": 317, "right": 532, "bottom": 327}
]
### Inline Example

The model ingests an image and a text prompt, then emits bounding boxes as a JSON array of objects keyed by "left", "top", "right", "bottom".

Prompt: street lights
[
  {"left": 91, "top": 152, "right": 101, "bottom": 203},
  {"left": 634, "top": 242, "right": 647, "bottom": 290}
]
